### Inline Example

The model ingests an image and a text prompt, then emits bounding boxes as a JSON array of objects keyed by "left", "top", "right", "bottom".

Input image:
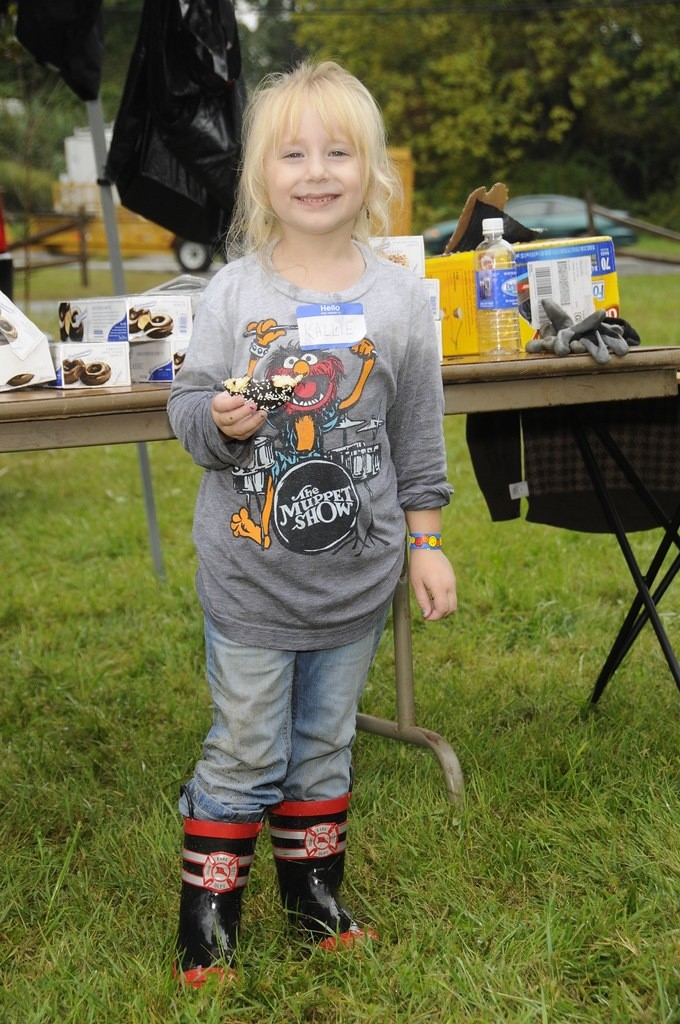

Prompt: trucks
[{"left": 28, "top": 119, "right": 416, "bottom": 274}]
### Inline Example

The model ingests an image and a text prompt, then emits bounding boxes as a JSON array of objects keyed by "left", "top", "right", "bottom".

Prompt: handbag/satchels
[{"left": 97, "top": 0, "right": 243, "bottom": 245}]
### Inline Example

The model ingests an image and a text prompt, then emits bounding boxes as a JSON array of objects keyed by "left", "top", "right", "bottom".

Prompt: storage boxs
[{"left": 0, "top": 183, "right": 620, "bottom": 393}]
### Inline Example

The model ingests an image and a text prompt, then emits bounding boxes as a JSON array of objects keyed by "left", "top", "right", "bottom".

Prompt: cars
[{"left": 420, "top": 193, "right": 641, "bottom": 255}]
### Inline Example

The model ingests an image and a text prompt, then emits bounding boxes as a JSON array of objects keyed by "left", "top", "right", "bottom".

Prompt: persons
[{"left": 164, "top": 62, "right": 459, "bottom": 989}]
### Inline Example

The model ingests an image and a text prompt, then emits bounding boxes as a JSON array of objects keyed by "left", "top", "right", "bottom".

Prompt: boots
[
  {"left": 268, "top": 793, "right": 381, "bottom": 956},
  {"left": 172, "top": 816, "right": 264, "bottom": 994}
]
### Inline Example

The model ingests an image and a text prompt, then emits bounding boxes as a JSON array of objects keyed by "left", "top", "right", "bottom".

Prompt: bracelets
[{"left": 409, "top": 533, "right": 442, "bottom": 550}]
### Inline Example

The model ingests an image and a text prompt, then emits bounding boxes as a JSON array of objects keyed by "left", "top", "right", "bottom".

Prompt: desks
[{"left": 0, "top": 345, "right": 680, "bottom": 812}]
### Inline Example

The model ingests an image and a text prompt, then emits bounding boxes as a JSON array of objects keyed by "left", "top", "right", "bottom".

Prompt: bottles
[{"left": 473, "top": 217, "right": 522, "bottom": 359}]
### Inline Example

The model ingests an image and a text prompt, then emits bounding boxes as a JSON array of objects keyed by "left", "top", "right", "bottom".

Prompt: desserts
[
  {"left": 59, "top": 303, "right": 70, "bottom": 341},
  {"left": 223, "top": 375, "right": 302, "bottom": 412},
  {"left": 129, "top": 307, "right": 151, "bottom": 333},
  {"left": 63, "top": 358, "right": 85, "bottom": 384},
  {"left": 144, "top": 314, "right": 174, "bottom": 338},
  {"left": 80, "top": 362, "right": 111, "bottom": 385},
  {"left": 69, "top": 307, "right": 83, "bottom": 341}
]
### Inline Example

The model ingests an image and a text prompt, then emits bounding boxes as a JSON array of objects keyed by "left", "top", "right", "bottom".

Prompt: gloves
[{"left": 525, "top": 298, "right": 641, "bottom": 364}]
